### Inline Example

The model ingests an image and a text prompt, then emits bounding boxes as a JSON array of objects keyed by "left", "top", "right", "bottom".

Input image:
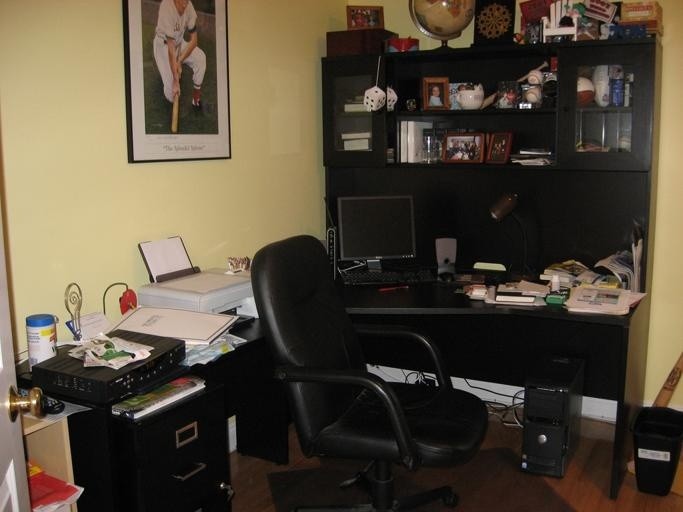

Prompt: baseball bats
[
  {"left": 480, "top": 61, "right": 549, "bottom": 110},
  {"left": 171, "top": 44, "right": 180, "bottom": 133}
]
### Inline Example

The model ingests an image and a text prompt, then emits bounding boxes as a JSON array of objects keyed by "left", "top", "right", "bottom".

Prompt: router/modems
[{"left": 326, "top": 225, "right": 337, "bottom": 281}]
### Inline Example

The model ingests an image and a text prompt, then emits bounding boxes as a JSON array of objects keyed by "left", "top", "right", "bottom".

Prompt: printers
[{"left": 138, "top": 235, "right": 260, "bottom": 319}]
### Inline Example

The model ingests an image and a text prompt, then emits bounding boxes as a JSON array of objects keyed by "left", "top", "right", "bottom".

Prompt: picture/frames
[
  {"left": 442, "top": 133, "right": 486, "bottom": 163},
  {"left": 121, "top": 1, "right": 231, "bottom": 164},
  {"left": 422, "top": 77, "right": 450, "bottom": 110},
  {"left": 483, "top": 132, "right": 513, "bottom": 165}
]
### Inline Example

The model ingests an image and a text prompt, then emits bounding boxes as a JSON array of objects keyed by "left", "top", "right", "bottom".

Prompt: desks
[{"left": 20, "top": 317, "right": 291, "bottom": 511}]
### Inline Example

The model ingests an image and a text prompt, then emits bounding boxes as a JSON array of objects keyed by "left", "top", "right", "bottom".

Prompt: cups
[
  {"left": 487, "top": 286, "right": 496, "bottom": 301},
  {"left": 455, "top": 90, "right": 484, "bottom": 111}
]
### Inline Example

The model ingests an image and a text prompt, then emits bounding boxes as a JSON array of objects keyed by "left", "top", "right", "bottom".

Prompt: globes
[{"left": 409, "top": 0, "right": 476, "bottom": 50}]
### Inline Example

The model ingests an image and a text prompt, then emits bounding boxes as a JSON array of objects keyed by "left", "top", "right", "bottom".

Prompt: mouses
[{"left": 439, "top": 272, "right": 455, "bottom": 283}]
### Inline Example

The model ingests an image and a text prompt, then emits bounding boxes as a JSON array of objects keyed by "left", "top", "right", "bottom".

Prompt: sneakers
[{"left": 191, "top": 91, "right": 202, "bottom": 110}]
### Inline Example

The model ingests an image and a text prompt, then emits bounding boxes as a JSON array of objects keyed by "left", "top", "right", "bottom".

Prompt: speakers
[{"left": 436, "top": 238, "right": 457, "bottom": 274}]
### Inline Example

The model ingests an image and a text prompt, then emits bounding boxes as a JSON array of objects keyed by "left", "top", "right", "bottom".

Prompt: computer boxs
[{"left": 520, "top": 358, "right": 585, "bottom": 478}]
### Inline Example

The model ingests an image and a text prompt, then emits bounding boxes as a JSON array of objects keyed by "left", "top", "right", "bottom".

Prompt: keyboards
[{"left": 338, "top": 269, "right": 436, "bottom": 286}]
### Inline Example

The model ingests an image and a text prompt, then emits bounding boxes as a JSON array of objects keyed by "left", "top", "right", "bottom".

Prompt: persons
[
  {"left": 447, "top": 135, "right": 480, "bottom": 161},
  {"left": 151, "top": 1, "right": 206, "bottom": 113},
  {"left": 428, "top": 82, "right": 443, "bottom": 106},
  {"left": 350, "top": 8, "right": 376, "bottom": 26},
  {"left": 494, "top": 139, "right": 502, "bottom": 161}
]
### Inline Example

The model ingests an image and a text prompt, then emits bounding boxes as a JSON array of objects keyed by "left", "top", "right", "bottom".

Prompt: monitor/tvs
[{"left": 337, "top": 195, "right": 417, "bottom": 261}]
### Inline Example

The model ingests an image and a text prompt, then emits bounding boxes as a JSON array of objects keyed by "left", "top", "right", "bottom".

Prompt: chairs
[{"left": 250, "top": 235, "right": 488, "bottom": 512}]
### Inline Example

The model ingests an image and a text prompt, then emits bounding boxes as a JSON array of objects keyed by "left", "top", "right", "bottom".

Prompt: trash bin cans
[{"left": 630, "top": 406, "right": 683, "bottom": 497}]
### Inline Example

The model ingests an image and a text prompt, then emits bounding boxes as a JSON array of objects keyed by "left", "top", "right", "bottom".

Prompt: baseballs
[
  {"left": 528, "top": 70, "right": 543, "bottom": 85},
  {"left": 525, "top": 85, "right": 542, "bottom": 103}
]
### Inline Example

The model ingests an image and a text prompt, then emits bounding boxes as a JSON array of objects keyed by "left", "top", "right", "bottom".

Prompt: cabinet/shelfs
[
  {"left": 21, "top": 404, "right": 79, "bottom": 511},
  {"left": 322, "top": 36, "right": 661, "bottom": 500},
  {"left": 69, "top": 381, "right": 234, "bottom": 510}
]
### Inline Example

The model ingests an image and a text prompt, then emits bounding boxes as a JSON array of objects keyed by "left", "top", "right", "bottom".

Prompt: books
[
  {"left": 471, "top": 238, "right": 643, "bottom": 316},
  {"left": 111, "top": 373, "right": 205, "bottom": 420}
]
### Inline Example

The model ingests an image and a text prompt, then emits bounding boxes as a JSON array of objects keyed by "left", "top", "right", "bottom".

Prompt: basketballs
[{"left": 577, "top": 77, "right": 595, "bottom": 106}]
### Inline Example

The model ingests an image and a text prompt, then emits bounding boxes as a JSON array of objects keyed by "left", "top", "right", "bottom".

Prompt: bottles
[
  {"left": 497, "top": 82, "right": 543, "bottom": 109},
  {"left": 621, "top": 275, "right": 628, "bottom": 289},
  {"left": 423, "top": 129, "right": 436, "bottom": 163},
  {"left": 386, "top": 146, "right": 394, "bottom": 163},
  {"left": 25, "top": 314, "right": 56, "bottom": 371},
  {"left": 550, "top": 274, "right": 560, "bottom": 292},
  {"left": 609, "top": 65, "right": 634, "bottom": 109}
]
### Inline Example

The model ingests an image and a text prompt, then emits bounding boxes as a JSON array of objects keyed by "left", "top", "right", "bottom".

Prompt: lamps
[{"left": 488, "top": 191, "right": 537, "bottom": 282}]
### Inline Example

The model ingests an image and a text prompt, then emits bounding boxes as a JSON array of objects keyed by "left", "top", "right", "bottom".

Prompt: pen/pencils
[
  {"left": 378, "top": 285, "right": 410, "bottom": 292},
  {"left": 228, "top": 256, "right": 251, "bottom": 273}
]
